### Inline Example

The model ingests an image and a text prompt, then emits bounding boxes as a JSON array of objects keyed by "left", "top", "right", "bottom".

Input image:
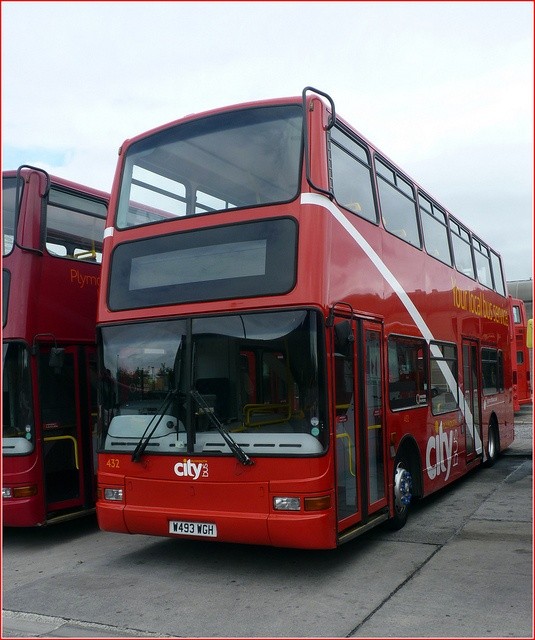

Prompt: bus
[
  {"left": 506, "top": 294, "right": 520, "bottom": 411},
  {"left": 95, "top": 86, "right": 515, "bottom": 550},
  {"left": 525, "top": 317, "right": 534, "bottom": 351},
  {"left": 513, "top": 295, "right": 532, "bottom": 406},
  {"left": 1, "top": 165, "right": 181, "bottom": 528}
]
